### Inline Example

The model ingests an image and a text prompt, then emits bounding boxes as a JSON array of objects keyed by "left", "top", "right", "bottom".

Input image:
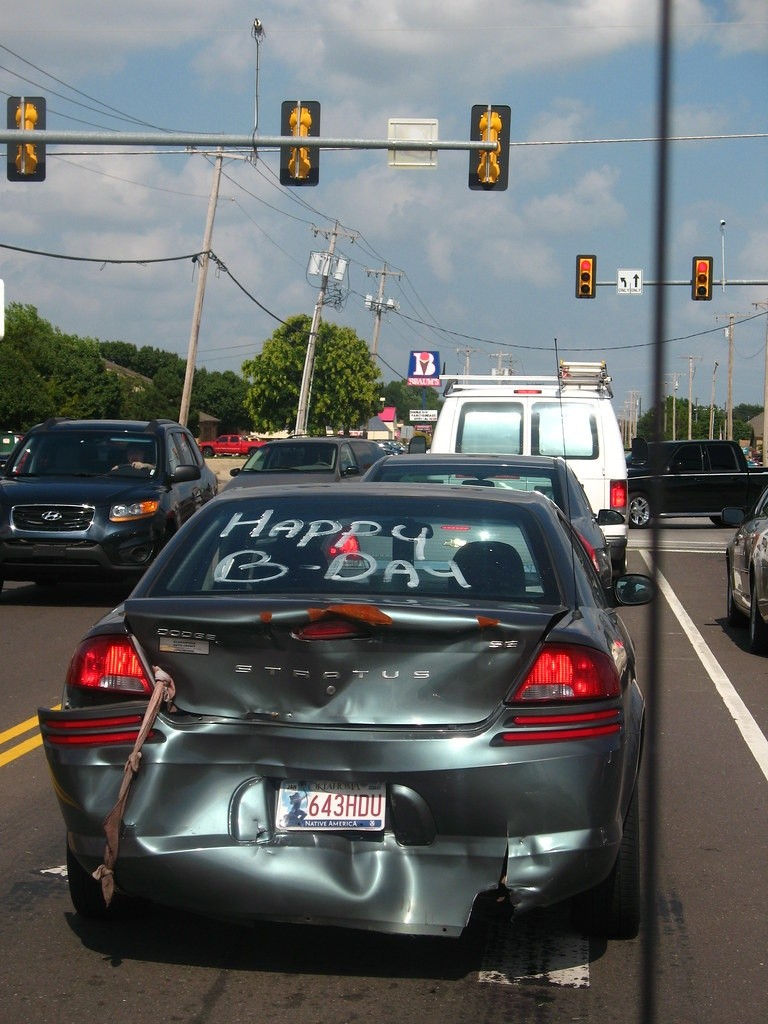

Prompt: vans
[{"left": 424, "top": 362, "right": 632, "bottom": 597}]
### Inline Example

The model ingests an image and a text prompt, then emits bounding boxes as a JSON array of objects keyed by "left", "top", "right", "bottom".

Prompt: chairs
[
  {"left": 254, "top": 535, "right": 334, "bottom": 578},
  {"left": 447, "top": 540, "right": 526, "bottom": 593}
]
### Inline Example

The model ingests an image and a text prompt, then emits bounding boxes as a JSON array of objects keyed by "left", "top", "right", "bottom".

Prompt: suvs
[{"left": 0, "top": 414, "right": 221, "bottom": 602}]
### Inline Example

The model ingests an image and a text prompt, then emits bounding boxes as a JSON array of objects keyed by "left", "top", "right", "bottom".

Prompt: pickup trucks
[
  {"left": 626, "top": 438, "right": 768, "bottom": 531},
  {"left": 196, "top": 433, "right": 268, "bottom": 458}
]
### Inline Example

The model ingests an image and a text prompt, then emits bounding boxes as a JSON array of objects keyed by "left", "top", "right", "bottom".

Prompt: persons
[{"left": 112, "top": 442, "right": 155, "bottom": 471}]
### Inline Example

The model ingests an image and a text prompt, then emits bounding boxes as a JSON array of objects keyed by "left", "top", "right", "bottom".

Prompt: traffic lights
[
  {"left": 576, "top": 255, "right": 597, "bottom": 298},
  {"left": 690, "top": 256, "right": 716, "bottom": 301}
]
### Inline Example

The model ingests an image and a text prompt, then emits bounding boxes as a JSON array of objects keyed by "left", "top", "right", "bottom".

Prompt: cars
[
  {"left": 380, "top": 440, "right": 413, "bottom": 459},
  {"left": 216, "top": 434, "right": 392, "bottom": 495},
  {"left": 720, "top": 485, "right": 768, "bottom": 651},
  {"left": 321, "top": 447, "right": 659, "bottom": 611},
  {"left": 0, "top": 432, "right": 23, "bottom": 478},
  {"left": 34, "top": 484, "right": 657, "bottom": 946}
]
